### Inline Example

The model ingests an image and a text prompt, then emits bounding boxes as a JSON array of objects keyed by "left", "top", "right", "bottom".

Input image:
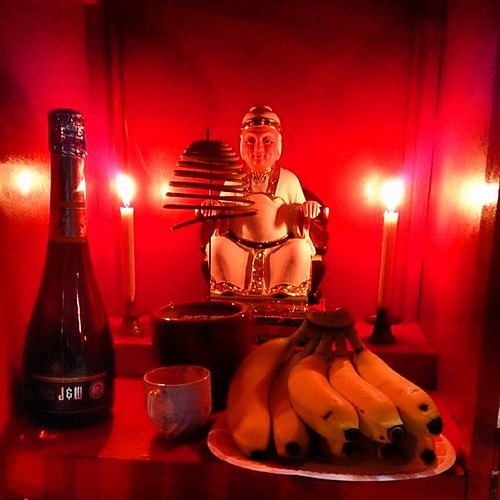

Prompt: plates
[{"left": 208, "top": 427, "right": 456, "bottom": 481}]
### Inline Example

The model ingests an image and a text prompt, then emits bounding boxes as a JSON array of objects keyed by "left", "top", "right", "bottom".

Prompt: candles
[
  {"left": 112, "top": 173, "right": 138, "bottom": 302},
  {"left": 376, "top": 178, "right": 405, "bottom": 309}
]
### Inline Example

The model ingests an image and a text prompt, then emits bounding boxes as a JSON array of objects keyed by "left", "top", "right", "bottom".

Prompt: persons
[{"left": 202, "top": 105, "right": 323, "bottom": 302}]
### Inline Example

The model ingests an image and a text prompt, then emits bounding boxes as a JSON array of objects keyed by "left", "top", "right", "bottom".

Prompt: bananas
[{"left": 228, "top": 305, "right": 443, "bottom": 458}]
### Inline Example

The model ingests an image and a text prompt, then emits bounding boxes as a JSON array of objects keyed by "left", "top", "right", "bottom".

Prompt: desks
[{"left": 0, "top": 312, "right": 466, "bottom": 500}]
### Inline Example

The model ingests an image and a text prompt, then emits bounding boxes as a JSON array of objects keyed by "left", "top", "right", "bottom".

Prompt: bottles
[{"left": 22, "top": 110, "right": 116, "bottom": 426}]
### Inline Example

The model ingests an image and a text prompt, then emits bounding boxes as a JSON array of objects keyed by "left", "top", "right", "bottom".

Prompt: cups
[
  {"left": 153, "top": 301, "right": 252, "bottom": 407},
  {"left": 144, "top": 366, "right": 210, "bottom": 430}
]
